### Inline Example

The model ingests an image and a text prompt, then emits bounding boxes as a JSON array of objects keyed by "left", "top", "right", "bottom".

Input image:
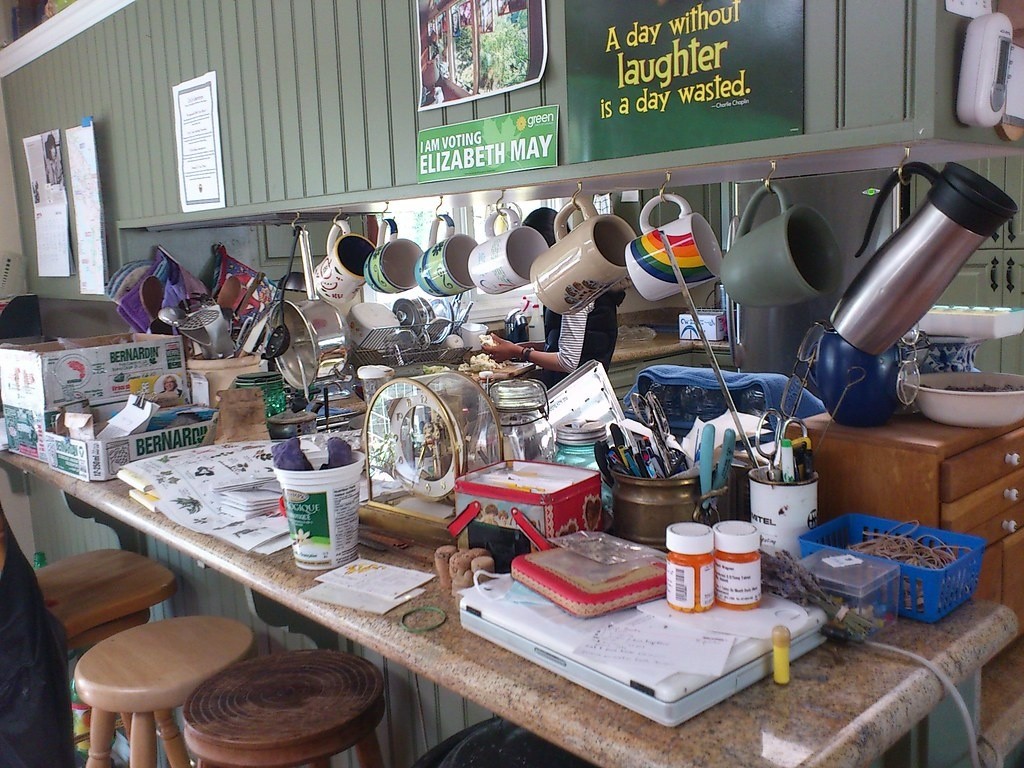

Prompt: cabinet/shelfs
[
  {"left": 795, "top": 409, "right": 1024, "bottom": 630},
  {"left": 908, "top": 152, "right": 1024, "bottom": 248},
  {"left": 908, "top": 251, "right": 1024, "bottom": 375}
]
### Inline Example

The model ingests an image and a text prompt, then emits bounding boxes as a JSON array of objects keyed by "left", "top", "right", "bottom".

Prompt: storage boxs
[
  {"left": 0, "top": 335, "right": 188, "bottom": 462},
  {"left": 44, "top": 410, "right": 223, "bottom": 486}
]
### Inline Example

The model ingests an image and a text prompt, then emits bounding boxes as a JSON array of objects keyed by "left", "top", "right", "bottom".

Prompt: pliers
[{"left": 699, "top": 424, "right": 737, "bottom": 528}]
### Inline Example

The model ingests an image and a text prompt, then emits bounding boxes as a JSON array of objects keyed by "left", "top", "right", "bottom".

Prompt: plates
[{"left": 393, "top": 298, "right": 454, "bottom": 345}]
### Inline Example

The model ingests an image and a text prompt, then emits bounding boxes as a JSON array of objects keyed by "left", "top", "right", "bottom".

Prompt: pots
[{"left": 268, "top": 224, "right": 349, "bottom": 390}]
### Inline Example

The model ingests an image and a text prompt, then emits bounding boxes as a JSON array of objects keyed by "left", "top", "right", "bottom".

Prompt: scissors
[
  {"left": 630, "top": 390, "right": 672, "bottom": 474},
  {"left": 755, "top": 408, "right": 807, "bottom": 482}
]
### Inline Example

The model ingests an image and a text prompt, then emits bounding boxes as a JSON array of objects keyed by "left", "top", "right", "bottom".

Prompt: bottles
[
  {"left": 665, "top": 521, "right": 761, "bottom": 614},
  {"left": 234, "top": 371, "right": 288, "bottom": 432},
  {"left": 488, "top": 378, "right": 613, "bottom": 513},
  {"left": 31, "top": 551, "right": 117, "bottom": 762}
]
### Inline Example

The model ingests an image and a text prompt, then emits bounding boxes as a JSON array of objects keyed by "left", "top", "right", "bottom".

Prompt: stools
[
  {"left": 182, "top": 649, "right": 386, "bottom": 768},
  {"left": 414, "top": 716, "right": 594, "bottom": 767},
  {"left": 74, "top": 617, "right": 254, "bottom": 768},
  {"left": 32, "top": 545, "right": 175, "bottom": 634}
]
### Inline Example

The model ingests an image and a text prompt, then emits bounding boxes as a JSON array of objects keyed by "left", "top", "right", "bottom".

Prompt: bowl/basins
[
  {"left": 345, "top": 302, "right": 401, "bottom": 350},
  {"left": 906, "top": 371, "right": 1024, "bottom": 428}
]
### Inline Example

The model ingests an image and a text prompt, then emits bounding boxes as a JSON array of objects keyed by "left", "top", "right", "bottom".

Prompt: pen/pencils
[
  {"left": 601, "top": 423, "right": 671, "bottom": 477},
  {"left": 667, "top": 453, "right": 685, "bottom": 477}
]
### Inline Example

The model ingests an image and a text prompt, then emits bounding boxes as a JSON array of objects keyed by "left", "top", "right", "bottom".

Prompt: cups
[
  {"left": 363, "top": 218, "right": 425, "bottom": 295},
  {"left": 460, "top": 322, "right": 488, "bottom": 352},
  {"left": 747, "top": 464, "right": 820, "bottom": 560},
  {"left": 624, "top": 193, "right": 723, "bottom": 302},
  {"left": 272, "top": 450, "right": 366, "bottom": 571},
  {"left": 414, "top": 214, "right": 479, "bottom": 297},
  {"left": 313, "top": 219, "right": 375, "bottom": 304},
  {"left": 830, "top": 160, "right": 1020, "bottom": 357},
  {"left": 720, "top": 182, "right": 844, "bottom": 310},
  {"left": 467, "top": 208, "right": 550, "bottom": 295},
  {"left": 529, "top": 196, "right": 638, "bottom": 316}
]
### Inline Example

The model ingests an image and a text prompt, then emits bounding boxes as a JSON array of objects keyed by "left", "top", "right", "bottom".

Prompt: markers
[{"left": 780, "top": 438, "right": 794, "bottom": 483}]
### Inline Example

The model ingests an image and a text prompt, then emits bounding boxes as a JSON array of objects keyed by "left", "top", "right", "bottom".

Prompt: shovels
[
  {"left": 139, "top": 275, "right": 164, "bottom": 323},
  {"left": 177, "top": 310, "right": 219, "bottom": 330}
]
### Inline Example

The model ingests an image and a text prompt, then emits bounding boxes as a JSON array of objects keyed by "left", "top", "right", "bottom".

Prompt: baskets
[{"left": 800, "top": 512, "right": 986, "bottom": 623}]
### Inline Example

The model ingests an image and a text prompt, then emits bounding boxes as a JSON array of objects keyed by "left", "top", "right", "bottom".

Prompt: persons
[
  {"left": 45, "top": 135, "right": 64, "bottom": 184},
  {"left": 481, "top": 207, "right": 626, "bottom": 390},
  {"left": 160, "top": 375, "right": 182, "bottom": 398},
  {"left": 0, "top": 500, "right": 75, "bottom": 768}
]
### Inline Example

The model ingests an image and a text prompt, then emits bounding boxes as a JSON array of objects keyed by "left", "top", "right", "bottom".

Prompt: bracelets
[{"left": 522, "top": 346, "right": 535, "bottom": 361}]
